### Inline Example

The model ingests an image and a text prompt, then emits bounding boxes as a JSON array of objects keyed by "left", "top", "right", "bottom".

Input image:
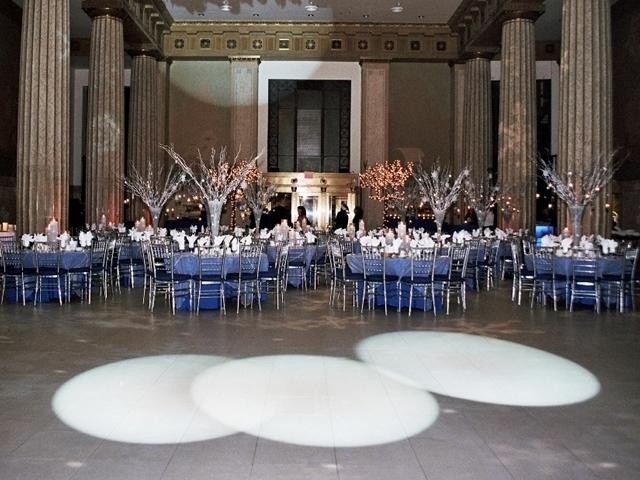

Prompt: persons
[
  {"left": 337, "top": 204, "right": 349, "bottom": 229},
  {"left": 353, "top": 206, "right": 362, "bottom": 233},
  {"left": 296, "top": 205, "right": 306, "bottom": 231}
]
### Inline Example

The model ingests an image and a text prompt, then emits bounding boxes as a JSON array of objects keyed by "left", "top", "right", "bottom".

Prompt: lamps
[
  {"left": 390, "top": 1, "right": 405, "bottom": 14},
  {"left": 219, "top": 0, "right": 232, "bottom": 13},
  {"left": 304, "top": 0, "right": 318, "bottom": 13}
]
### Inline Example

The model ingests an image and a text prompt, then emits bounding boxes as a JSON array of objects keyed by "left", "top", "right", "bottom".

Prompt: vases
[
  {"left": 567, "top": 206, "right": 585, "bottom": 247},
  {"left": 434, "top": 210, "right": 446, "bottom": 238},
  {"left": 150, "top": 207, "right": 161, "bottom": 235},
  {"left": 207, "top": 200, "right": 222, "bottom": 239},
  {"left": 253, "top": 209, "right": 263, "bottom": 234},
  {"left": 475, "top": 210, "right": 488, "bottom": 228}
]
